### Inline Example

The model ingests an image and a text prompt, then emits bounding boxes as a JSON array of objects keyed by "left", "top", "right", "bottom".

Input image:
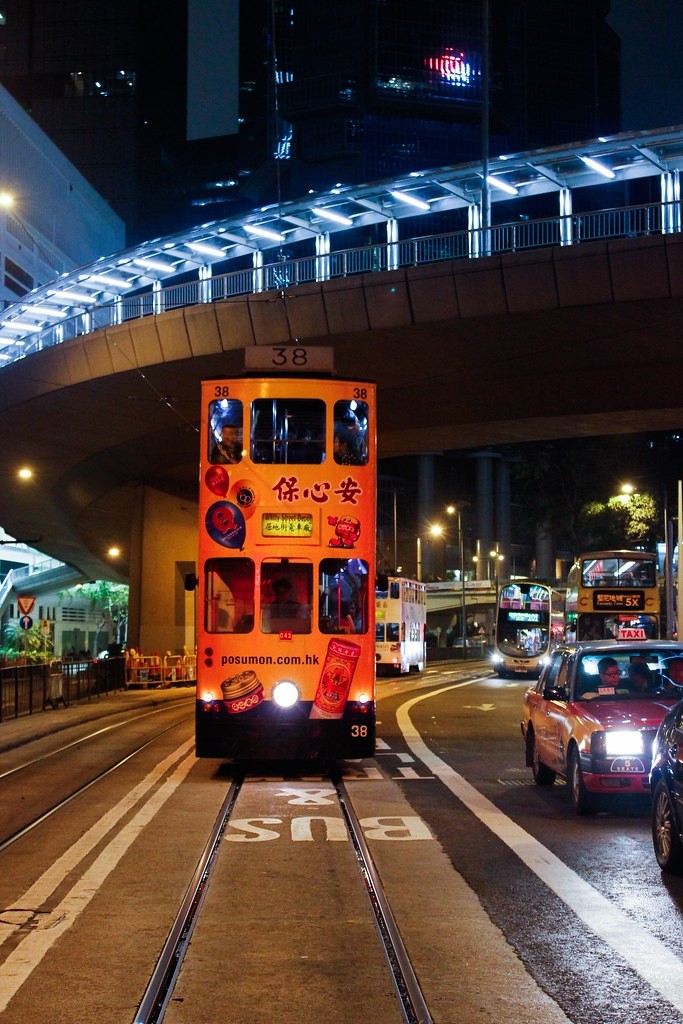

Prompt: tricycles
[{"left": 448, "top": 622, "right": 489, "bottom": 661}]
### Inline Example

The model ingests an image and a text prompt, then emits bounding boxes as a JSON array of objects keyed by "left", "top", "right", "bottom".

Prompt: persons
[
  {"left": 411, "top": 565, "right": 497, "bottom": 658},
  {"left": 252, "top": 399, "right": 326, "bottom": 464},
  {"left": 40, "top": 640, "right": 139, "bottom": 692},
  {"left": 658, "top": 539, "right": 680, "bottom": 637},
  {"left": 318, "top": 558, "right": 367, "bottom": 635},
  {"left": 374, "top": 579, "right": 425, "bottom": 643},
  {"left": 577, "top": 559, "right": 657, "bottom": 641},
  {"left": 211, "top": 425, "right": 243, "bottom": 465},
  {"left": 333, "top": 398, "right": 370, "bottom": 466},
  {"left": 577, "top": 658, "right": 628, "bottom": 702},
  {"left": 624, "top": 662, "right": 668, "bottom": 698},
  {"left": 261, "top": 577, "right": 311, "bottom": 633},
  {"left": 497, "top": 584, "right": 565, "bottom": 660}
]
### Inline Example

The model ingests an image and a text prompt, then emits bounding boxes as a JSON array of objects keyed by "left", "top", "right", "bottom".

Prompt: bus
[
  {"left": 493, "top": 581, "right": 567, "bottom": 678},
  {"left": 373, "top": 575, "right": 426, "bottom": 675},
  {"left": 568, "top": 549, "right": 660, "bottom": 642},
  {"left": 194, "top": 379, "right": 377, "bottom": 768}
]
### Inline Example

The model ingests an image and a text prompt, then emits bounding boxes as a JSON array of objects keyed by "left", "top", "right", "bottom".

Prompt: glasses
[{"left": 603, "top": 670, "right": 621, "bottom": 677}]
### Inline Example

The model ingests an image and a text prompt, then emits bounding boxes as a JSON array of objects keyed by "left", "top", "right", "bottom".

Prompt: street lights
[
  {"left": 446, "top": 506, "right": 467, "bottom": 660},
  {"left": 618, "top": 470, "right": 675, "bottom": 638}
]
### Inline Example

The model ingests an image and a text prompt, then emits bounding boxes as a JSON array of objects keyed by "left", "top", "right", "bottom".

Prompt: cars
[
  {"left": 53, "top": 642, "right": 126, "bottom": 678},
  {"left": 519, "top": 628, "right": 683, "bottom": 816},
  {"left": 648, "top": 695, "right": 683, "bottom": 869}
]
